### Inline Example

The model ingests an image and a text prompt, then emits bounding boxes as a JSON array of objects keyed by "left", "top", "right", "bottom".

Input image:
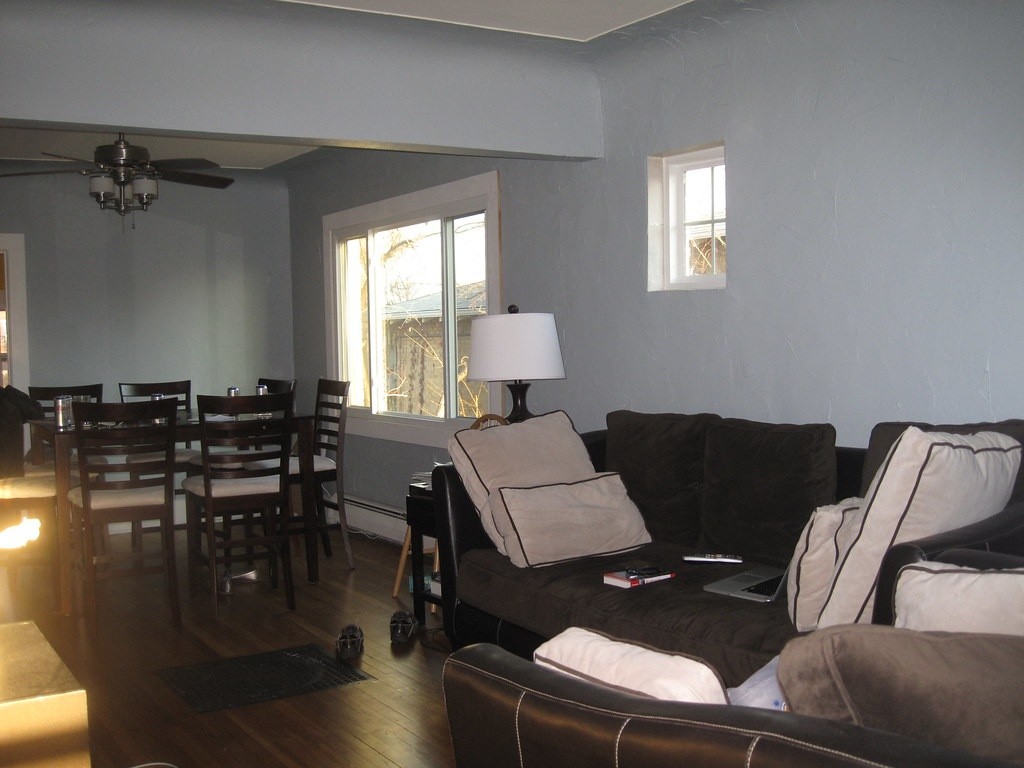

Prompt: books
[{"left": 604, "top": 569, "right": 676, "bottom": 589}]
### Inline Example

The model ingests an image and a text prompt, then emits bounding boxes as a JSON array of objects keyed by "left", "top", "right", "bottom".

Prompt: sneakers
[
  {"left": 336, "top": 620, "right": 367, "bottom": 664},
  {"left": 390, "top": 613, "right": 420, "bottom": 643}
]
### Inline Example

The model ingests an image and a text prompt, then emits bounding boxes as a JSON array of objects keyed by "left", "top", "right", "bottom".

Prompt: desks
[
  {"left": 28, "top": 408, "right": 319, "bottom": 616},
  {"left": 405, "top": 484, "right": 436, "bottom": 628}
]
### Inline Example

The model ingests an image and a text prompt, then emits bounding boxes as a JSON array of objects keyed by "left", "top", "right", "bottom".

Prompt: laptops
[{"left": 702, "top": 556, "right": 793, "bottom": 602}]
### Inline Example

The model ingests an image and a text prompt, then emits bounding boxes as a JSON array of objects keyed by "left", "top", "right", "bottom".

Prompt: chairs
[{"left": 0, "top": 376, "right": 353, "bottom": 648}]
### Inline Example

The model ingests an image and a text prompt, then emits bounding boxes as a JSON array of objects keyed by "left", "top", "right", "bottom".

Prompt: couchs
[{"left": 432, "top": 429, "right": 1024, "bottom": 768}]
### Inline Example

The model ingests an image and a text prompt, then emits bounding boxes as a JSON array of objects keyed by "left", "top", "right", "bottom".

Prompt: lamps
[
  {"left": 88, "top": 166, "right": 158, "bottom": 217},
  {"left": 466, "top": 304, "right": 566, "bottom": 422}
]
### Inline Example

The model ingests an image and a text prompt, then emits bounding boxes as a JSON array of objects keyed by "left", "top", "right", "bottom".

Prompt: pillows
[{"left": 447, "top": 406, "right": 1024, "bottom": 768}]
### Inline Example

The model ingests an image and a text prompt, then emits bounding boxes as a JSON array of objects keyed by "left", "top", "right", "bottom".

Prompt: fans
[{"left": 0, "top": 133, "right": 234, "bottom": 189}]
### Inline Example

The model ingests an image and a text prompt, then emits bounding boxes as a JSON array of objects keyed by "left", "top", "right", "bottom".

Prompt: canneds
[
  {"left": 54, "top": 394, "right": 73, "bottom": 426},
  {"left": 150, "top": 393, "right": 165, "bottom": 424},
  {"left": 256, "top": 385, "right": 268, "bottom": 395},
  {"left": 227, "top": 387, "right": 239, "bottom": 416}
]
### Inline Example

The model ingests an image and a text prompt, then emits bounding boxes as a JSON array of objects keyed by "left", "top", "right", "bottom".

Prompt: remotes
[{"left": 682, "top": 554, "right": 744, "bottom": 564}]
